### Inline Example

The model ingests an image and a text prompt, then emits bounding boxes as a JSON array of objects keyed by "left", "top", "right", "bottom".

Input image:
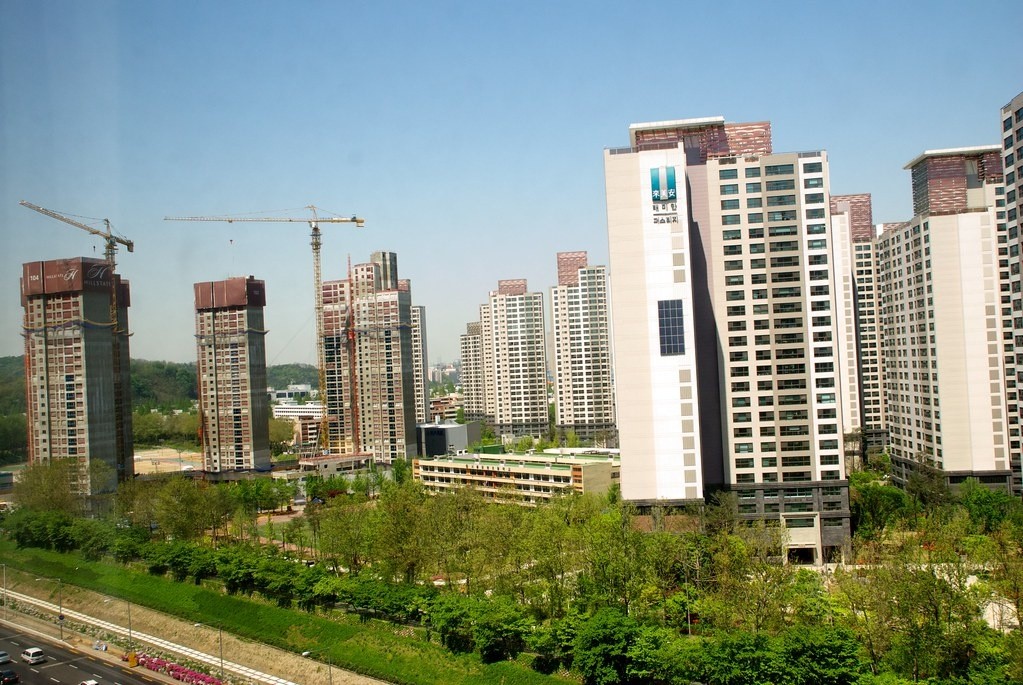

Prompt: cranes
[
  {"left": 163, "top": 204, "right": 367, "bottom": 455},
  {"left": 19, "top": 198, "right": 135, "bottom": 486}
]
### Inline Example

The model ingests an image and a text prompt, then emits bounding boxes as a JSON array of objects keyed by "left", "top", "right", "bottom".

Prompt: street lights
[
  {"left": 194, "top": 620, "right": 224, "bottom": 683},
  {"left": 104, "top": 598, "right": 133, "bottom": 651},
  {"left": 302, "top": 647, "right": 332, "bottom": 685},
  {"left": 36, "top": 578, "right": 63, "bottom": 640}
]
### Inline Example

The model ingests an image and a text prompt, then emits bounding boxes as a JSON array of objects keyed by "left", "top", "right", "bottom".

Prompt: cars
[
  {"left": 77, "top": 679, "right": 99, "bottom": 685},
  {"left": 0, "top": 651, "right": 12, "bottom": 664},
  {"left": 0, "top": 670, "right": 20, "bottom": 685}
]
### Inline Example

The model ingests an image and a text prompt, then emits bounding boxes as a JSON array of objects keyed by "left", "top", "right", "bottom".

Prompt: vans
[{"left": 21, "top": 647, "right": 44, "bottom": 666}]
